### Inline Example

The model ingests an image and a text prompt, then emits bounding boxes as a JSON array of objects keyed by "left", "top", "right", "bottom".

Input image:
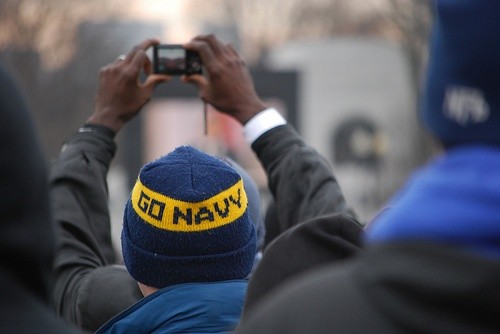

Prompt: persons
[
  {"left": 238, "top": 0, "right": 500, "bottom": 334},
  {"left": 0, "top": 35, "right": 363, "bottom": 334}
]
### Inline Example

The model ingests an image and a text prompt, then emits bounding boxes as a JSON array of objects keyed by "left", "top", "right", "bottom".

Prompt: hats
[
  {"left": 210, "top": 152, "right": 266, "bottom": 251},
  {"left": 120, "top": 144, "right": 256, "bottom": 289},
  {"left": 422, "top": 0, "right": 500, "bottom": 143}
]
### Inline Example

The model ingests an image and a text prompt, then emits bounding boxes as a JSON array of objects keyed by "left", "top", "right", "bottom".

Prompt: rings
[{"left": 118, "top": 54, "right": 126, "bottom": 60}]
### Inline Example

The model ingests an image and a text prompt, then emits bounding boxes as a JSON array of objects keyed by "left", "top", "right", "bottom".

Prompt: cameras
[{"left": 153, "top": 44, "right": 203, "bottom": 74}]
